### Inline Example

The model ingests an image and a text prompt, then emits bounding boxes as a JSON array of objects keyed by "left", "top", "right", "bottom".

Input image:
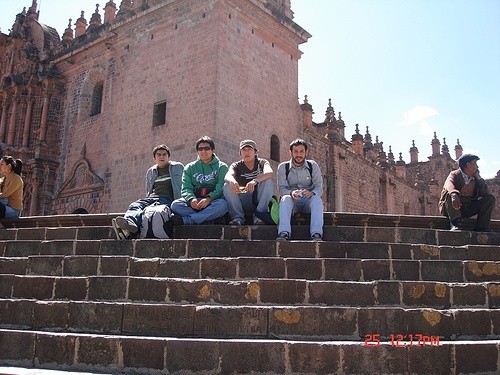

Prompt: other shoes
[
  {"left": 171, "top": 214, "right": 184, "bottom": 225},
  {"left": 111, "top": 218, "right": 129, "bottom": 239},
  {"left": 474, "top": 227, "right": 491, "bottom": 232},
  {"left": 253, "top": 214, "right": 265, "bottom": 225},
  {"left": 229, "top": 219, "right": 243, "bottom": 226},
  {"left": 451, "top": 225, "right": 463, "bottom": 230},
  {"left": 116, "top": 216, "right": 138, "bottom": 233}
]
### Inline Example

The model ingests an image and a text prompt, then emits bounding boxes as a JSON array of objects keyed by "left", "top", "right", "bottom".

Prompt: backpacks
[{"left": 139, "top": 202, "right": 172, "bottom": 240}]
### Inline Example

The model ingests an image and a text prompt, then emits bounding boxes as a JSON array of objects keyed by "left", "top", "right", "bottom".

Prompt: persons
[
  {"left": 438, "top": 153, "right": 496, "bottom": 233},
  {"left": 276, "top": 138, "right": 324, "bottom": 241},
  {"left": 0, "top": 155, "right": 24, "bottom": 220},
  {"left": 112, "top": 144, "right": 184, "bottom": 240},
  {"left": 222, "top": 139, "right": 274, "bottom": 225},
  {"left": 171, "top": 136, "right": 228, "bottom": 226}
]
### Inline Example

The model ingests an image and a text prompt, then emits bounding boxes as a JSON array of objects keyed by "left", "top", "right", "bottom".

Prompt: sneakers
[
  {"left": 276, "top": 231, "right": 292, "bottom": 242},
  {"left": 311, "top": 232, "right": 323, "bottom": 242}
]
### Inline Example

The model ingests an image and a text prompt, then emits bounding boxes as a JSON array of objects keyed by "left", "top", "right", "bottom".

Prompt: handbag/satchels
[{"left": 256, "top": 195, "right": 280, "bottom": 226}]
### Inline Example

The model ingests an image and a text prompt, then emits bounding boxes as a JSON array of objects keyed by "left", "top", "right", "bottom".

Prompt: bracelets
[{"left": 253, "top": 179, "right": 258, "bottom": 183}]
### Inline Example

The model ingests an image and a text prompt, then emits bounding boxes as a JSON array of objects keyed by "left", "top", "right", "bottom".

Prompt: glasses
[{"left": 197, "top": 146, "right": 213, "bottom": 151}]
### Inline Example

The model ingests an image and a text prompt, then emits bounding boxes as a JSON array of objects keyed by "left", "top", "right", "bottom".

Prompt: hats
[
  {"left": 239, "top": 140, "right": 257, "bottom": 151},
  {"left": 458, "top": 154, "right": 480, "bottom": 166}
]
